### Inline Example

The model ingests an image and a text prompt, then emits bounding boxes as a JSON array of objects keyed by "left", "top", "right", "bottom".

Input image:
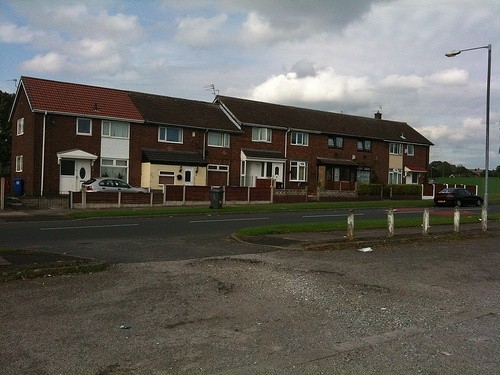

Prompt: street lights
[{"left": 445, "top": 43, "right": 492, "bottom": 232}]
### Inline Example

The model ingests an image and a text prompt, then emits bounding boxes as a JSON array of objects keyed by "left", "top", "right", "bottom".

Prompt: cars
[
  {"left": 434, "top": 187, "right": 484, "bottom": 208},
  {"left": 81, "top": 176, "right": 148, "bottom": 193}
]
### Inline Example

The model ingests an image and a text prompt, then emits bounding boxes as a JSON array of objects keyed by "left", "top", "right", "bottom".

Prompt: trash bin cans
[
  {"left": 209, "top": 189, "right": 224, "bottom": 209},
  {"left": 6, "top": 176, "right": 24, "bottom": 196}
]
